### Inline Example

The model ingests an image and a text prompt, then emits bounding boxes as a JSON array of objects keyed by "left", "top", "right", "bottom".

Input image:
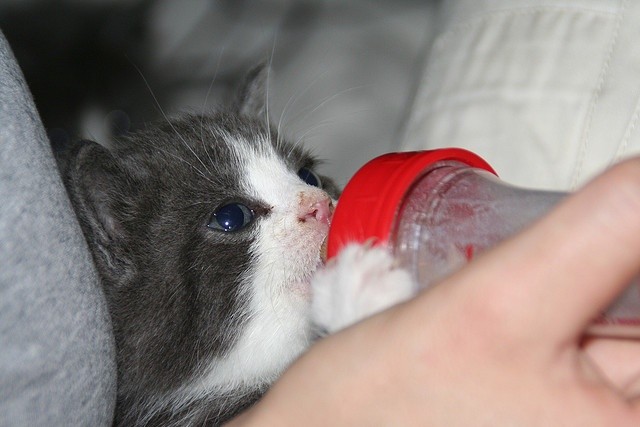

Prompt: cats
[{"left": 52, "top": 30, "right": 417, "bottom": 427}]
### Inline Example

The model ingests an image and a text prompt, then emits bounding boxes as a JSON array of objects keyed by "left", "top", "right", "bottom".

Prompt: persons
[{"left": 0, "top": 28, "right": 638, "bottom": 427}]
